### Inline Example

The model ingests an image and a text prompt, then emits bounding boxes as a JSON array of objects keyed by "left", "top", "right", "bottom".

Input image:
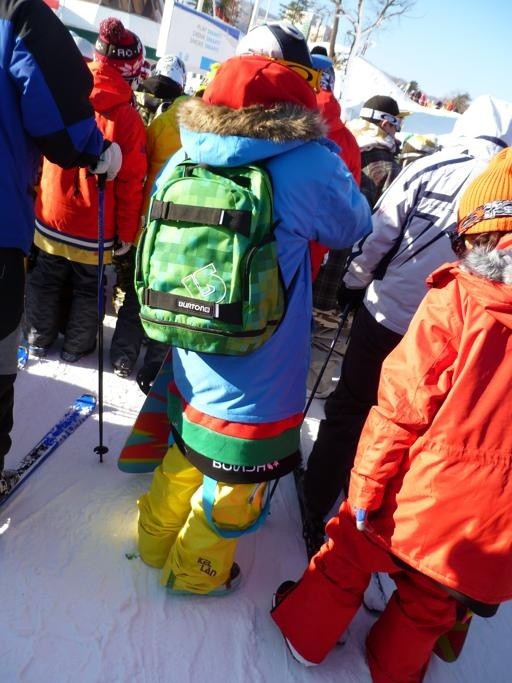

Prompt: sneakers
[
  {"left": 114, "top": 364, "right": 133, "bottom": 377},
  {"left": 272, "top": 580, "right": 322, "bottom": 666},
  {"left": 166, "top": 561, "right": 242, "bottom": 597},
  {"left": 59, "top": 339, "right": 99, "bottom": 363},
  {"left": 29, "top": 345, "right": 50, "bottom": 357}
]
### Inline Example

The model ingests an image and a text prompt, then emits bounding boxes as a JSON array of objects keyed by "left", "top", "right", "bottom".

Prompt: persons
[
  {"left": 26, "top": 19, "right": 148, "bottom": 362},
  {"left": 109, "top": 21, "right": 512, "bottom": 683},
  {"left": 0, "top": 0, "right": 123, "bottom": 496}
]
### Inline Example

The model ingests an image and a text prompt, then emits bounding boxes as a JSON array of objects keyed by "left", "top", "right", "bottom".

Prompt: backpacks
[{"left": 132, "top": 149, "right": 300, "bottom": 357}]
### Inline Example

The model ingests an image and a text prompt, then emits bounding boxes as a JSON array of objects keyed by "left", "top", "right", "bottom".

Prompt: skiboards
[
  {"left": 1, "top": 345, "right": 97, "bottom": 508},
  {"left": 297, "top": 418, "right": 391, "bottom": 633}
]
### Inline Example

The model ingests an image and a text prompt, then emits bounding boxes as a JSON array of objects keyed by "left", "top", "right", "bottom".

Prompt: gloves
[
  {"left": 89, "top": 138, "right": 124, "bottom": 183},
  {"left": 337, "top": 283, "right": 366, "bottom": 313}
]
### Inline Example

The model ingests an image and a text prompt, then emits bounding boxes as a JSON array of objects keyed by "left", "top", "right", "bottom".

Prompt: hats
[
  {"left": 93, "top": 16, "right": 146, "bottom": 83},
  {"left": 155, "top": 55, "right": 187, "bottom": 87},
  {"left": 310, "top": 46, "right": 334, "bottom": 87},
  {"left": 456, "top": 146, "right": 512, "bottom": 236},
  {"left": 234, "top": 20, "right": 314, "bottom": 72},
  {"left": 361, "top": 94, "right": 400, "bottom": 122}
]
